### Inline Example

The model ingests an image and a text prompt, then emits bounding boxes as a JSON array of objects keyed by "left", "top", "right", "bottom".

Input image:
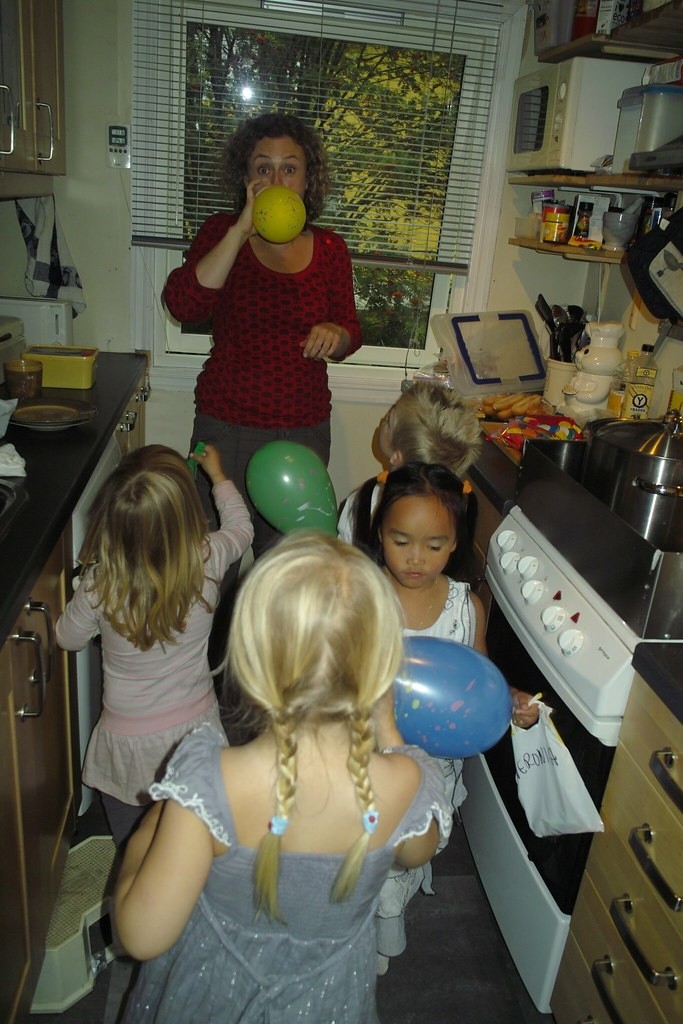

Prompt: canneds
[{"left": 538, "top": 204, "right": 571, "bottom": 244}]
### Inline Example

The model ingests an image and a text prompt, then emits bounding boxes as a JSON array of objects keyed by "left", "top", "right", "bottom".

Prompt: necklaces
[{"left": 418, "top": 579, "right": 437, "bottom": 630}]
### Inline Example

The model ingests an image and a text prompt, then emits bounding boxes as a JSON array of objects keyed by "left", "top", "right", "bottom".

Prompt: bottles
[
  {"left": 574, "top": 200, "right": 594, "bottom": 239},
  {"left": 607, "top": 344, "right": 658, "bottom": 421}
]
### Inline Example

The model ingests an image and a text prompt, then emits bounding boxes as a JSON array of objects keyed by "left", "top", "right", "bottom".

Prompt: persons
[
  {"left": 55, "top": 445, "right": 254, "bottom": 848},
  {"left": 163, "top": 114, "right": 362, "bottom": 671},
  {"left": 347, "top": 463, "right": 539, "bottom": 976},
  {"left": 116, "top": 530, "right": 454, "bottom": 1024}
]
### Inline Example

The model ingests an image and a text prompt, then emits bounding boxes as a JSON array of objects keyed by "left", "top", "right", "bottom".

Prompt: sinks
[{"left": 0, "top": 478, "right": 28, "bottom": 543}]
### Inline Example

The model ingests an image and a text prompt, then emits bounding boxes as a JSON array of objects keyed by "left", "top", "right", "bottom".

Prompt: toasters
[{"left": 0, "top": 294, "right": 74, "bottom": 352}]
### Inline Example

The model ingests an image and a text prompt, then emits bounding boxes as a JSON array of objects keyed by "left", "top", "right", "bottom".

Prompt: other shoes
[
  {"left": 377, "top": 916, "right": 407, "bottom": 955},
  {"left": 207, "top": 645, "right": 232, "bottom": 677}
]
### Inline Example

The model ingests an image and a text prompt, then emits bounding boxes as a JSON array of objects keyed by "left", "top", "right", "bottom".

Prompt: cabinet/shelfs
[
  {"left": 0, "top": 0, "right": 65, "bottom": 201},
  {"left": 507, "top": 0, "right": 683, "bottom": 265},
  {"left": 548, "top": 664, "right": 683, "bottom": 1023},
  {"left": 0, "top": 370, "right": 157, "bottom": 1022}
]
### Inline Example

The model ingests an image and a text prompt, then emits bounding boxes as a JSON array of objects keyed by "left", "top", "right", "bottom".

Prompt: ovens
[{"left": 457, "top": 566, "right": 619, "bottom": 1016}]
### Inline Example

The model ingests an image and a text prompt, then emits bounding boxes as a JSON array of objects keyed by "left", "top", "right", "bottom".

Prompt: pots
[{"left": 582, "top": 411, "right": 683, "bottom": 552}]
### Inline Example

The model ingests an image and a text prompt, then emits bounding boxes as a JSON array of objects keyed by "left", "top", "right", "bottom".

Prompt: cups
[
  {"left": 5, "top": 359, "right": 43, "bottom": 399},
  {"left": 542, "top": 358, "right": 580, "bottom": 407}
]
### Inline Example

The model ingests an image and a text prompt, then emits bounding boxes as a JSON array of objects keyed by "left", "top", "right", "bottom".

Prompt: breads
[{"left": 481, "top": 393, "right": 546, "bottom": 419}]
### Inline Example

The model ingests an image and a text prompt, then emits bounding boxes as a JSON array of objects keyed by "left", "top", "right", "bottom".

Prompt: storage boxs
[
  {"left": 524, "top": 0, "right": 633, "bottom": 55},
  {"left": 635, "top": 84, "right": 683, "bottom": 153},
  {"left": 19, "top": 344, "right": 99, "bottom": 388},
  {"left": 612, "top": 84, "right": 644, "bottom": 174}
]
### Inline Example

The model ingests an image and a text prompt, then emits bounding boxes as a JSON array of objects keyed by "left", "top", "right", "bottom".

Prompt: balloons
[
  {"left": 393, "top": 636, "right": 512, "bottom": 760},
  {"left": 187, "top": 442, "right": 206, "bottom": 476},
  {"left": 252, "top": 186, "right": 306, "bottom": 244},
  {"left": 246, "top": 440, "right": 339, "bottom": 538}
]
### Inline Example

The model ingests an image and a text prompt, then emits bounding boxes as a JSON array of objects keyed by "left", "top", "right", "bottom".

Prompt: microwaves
[{"left": 503, "top": 55, "right": 660, "bottom": 173}]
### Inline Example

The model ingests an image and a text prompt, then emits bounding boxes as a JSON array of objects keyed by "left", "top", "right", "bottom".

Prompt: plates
[{"left": 7, "top": 394, "right": 99, "bottom": 433}]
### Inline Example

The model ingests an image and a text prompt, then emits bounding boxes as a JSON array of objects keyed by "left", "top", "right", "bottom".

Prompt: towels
[
  {"left": 0, "top": 397, "right": 19, "bottom": 438},
  {"left": 14, "top": 193, "right": 87, "bottom": 320},
  {"left": 0, "top": 443, "right": 27, "bottom": 480}
]
